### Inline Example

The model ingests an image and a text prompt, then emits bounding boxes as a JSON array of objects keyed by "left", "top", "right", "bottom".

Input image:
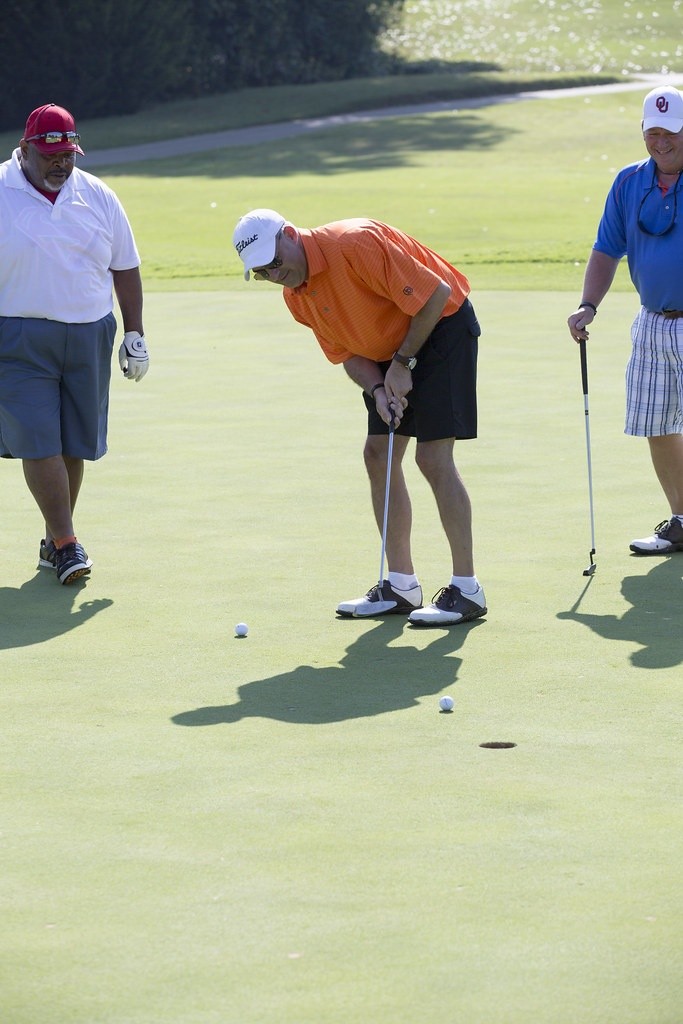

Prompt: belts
[{"left": 656, "top": 309, "right": 683, "bottom": 319}]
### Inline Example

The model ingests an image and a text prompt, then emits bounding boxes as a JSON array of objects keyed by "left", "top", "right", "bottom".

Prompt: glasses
[
  {"left": 25, "top": 131, "right": 81, "bottom": 145},
  {"left": 253, "top": 233, "right": 283, "bottom": 280},
  {"left": 637, "top": 190, "right": 677, "bottom": 237}
]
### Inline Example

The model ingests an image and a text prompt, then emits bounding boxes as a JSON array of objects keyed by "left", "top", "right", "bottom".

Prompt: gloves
[{"left": 119, "top": 331, "right": 149, "bottom": 382}]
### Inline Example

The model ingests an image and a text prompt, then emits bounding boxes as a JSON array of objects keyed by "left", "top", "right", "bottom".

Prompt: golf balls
[
  {"left": 235, "top": 623, "right": 249, "bottom": 635},
  {"left": 440, "top": 696, "right": 454, "bottom": 710}
]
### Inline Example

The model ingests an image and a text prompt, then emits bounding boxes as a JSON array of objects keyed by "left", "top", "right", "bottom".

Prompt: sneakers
[
  {"left": 38, "top": 539, "right": 94, "bottom": 584},
  {"left": 335, "top": 579, "right": 424, "bottom": 618},
  {"left": 407, "top": 582, "right": 487, "bottom": 627},
  {"left": 630, "top": 516, "right": 683, "bottom": 553}
]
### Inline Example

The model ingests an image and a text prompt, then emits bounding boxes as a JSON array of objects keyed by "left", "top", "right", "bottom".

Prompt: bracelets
[
  {"left": 371, "top": 382, "right": 385, "bottom": 399},
  {"left": 577, "top": 301, "right": 597, "bottom": 316}
]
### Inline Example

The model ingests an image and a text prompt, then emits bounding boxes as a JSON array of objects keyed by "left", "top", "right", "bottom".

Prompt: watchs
[{"left": 391, "top": 350, "right": 418, "bottom": 371}]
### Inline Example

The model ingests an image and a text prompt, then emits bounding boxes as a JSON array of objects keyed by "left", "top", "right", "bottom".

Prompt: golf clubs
[
  {"left": 579, "top": 326, "right": 596, "bottom": 576},
  {"left": 377, "top": 402, "right": 396, "bottom": 602}
]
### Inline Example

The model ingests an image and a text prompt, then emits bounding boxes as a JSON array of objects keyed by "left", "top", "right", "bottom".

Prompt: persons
[
  {"left": 0, "top": 102, "right": 150, "bottom": 588},
  {"left": 232, "top": 208, "right": 490, "bottom": 627},
  {"left": 566, "top": 85, "right": 683, "bottom": 557}
]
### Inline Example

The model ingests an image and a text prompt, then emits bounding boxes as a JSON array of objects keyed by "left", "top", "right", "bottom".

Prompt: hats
[
  {"left": 232, "top": 209, "right": 285, "bottom": 281},
  {"left": 24, "top": 103, "right": 84, "bottom": 156},
  {"left": 643, "top": 85, "right": 683, "bottom": 133}
]
